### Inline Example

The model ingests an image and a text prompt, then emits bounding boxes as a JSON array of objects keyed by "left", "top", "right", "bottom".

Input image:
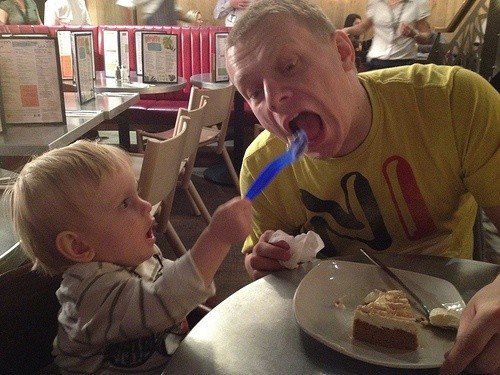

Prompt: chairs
[
  {"left": 189, "top": 73, "right": 246, "bottom": 187},
  {"left": 134, "top": 117, "right": 191, "bottom": 260},
  {"left": 128, "top": 95, "right": 214, "bottom": 227},
  {"left": 136, "top": 86, "right": 241, "bottom": 217}
]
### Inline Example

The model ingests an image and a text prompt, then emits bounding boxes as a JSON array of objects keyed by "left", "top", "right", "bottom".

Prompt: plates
[{"left": 293, "top": 258, "right": 471, "bottom": 369}]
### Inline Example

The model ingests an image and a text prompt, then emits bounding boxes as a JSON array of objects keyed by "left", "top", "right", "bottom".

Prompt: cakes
[{"left": 353, "top": 290, "right": 419, "bottom": 350}]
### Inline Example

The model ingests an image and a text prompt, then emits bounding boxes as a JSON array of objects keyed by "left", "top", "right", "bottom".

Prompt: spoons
[
  {"left": 358, "top": 247, "right": 462, "bottom": 330},
  {"left": 240, "top": 131, "right": 309, "bottom": 202}
]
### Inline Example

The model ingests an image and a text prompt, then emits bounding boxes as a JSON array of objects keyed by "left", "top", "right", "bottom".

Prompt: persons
[
  {"left": 10, "top": 139, "right": 252, "bottom": 375},
  {"left": 224, "top": 0, "right": 500, "bottom": 375},
  {"left": 351, "top": 39, "right": 367, "bottom": 73},
  {"left": 44, "top": 0, "right": 91, "bottom": 26},
  {"left": 341, "top": 0, "right": 432, "bottom": 72},
  {"left": 116, "top": 0, "right": 192, "bottom": 26},
  {"left": 343, "top": 14, "right": 365, "bottom": 40},
  {"left": 183, "top": 9, "right": 204, "bottom": 26},
  {"left": 0, "top": 0, "right": 43, "bottom": 25},
  {"left": 213, "top": 0, "right": 256, "bottom": 27}
]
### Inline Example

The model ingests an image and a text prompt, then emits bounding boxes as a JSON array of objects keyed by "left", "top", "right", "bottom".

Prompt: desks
[
  {"left": 161, "top": 254, "right": 500, "bottom": 375},
  {"left": 64, "top": 71, "right": 188, "bottom": 147},
  {"left": 62, "top": 90, "right": 141, "bottom": 130},
  {"left": 0, "top": 168, "right": 23, "bottom": 276},
  {"left": 0, "top": 110, "right": 105, "bottom": 158}
]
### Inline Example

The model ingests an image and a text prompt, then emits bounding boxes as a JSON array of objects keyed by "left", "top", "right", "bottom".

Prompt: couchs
[{"left": 0, "top": 24, "right": 313, "bottom": 132}]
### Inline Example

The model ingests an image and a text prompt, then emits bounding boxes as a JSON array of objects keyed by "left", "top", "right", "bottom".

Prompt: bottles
[{"left": 115, "top": 65, "right": 128, "bottom": 81}]
